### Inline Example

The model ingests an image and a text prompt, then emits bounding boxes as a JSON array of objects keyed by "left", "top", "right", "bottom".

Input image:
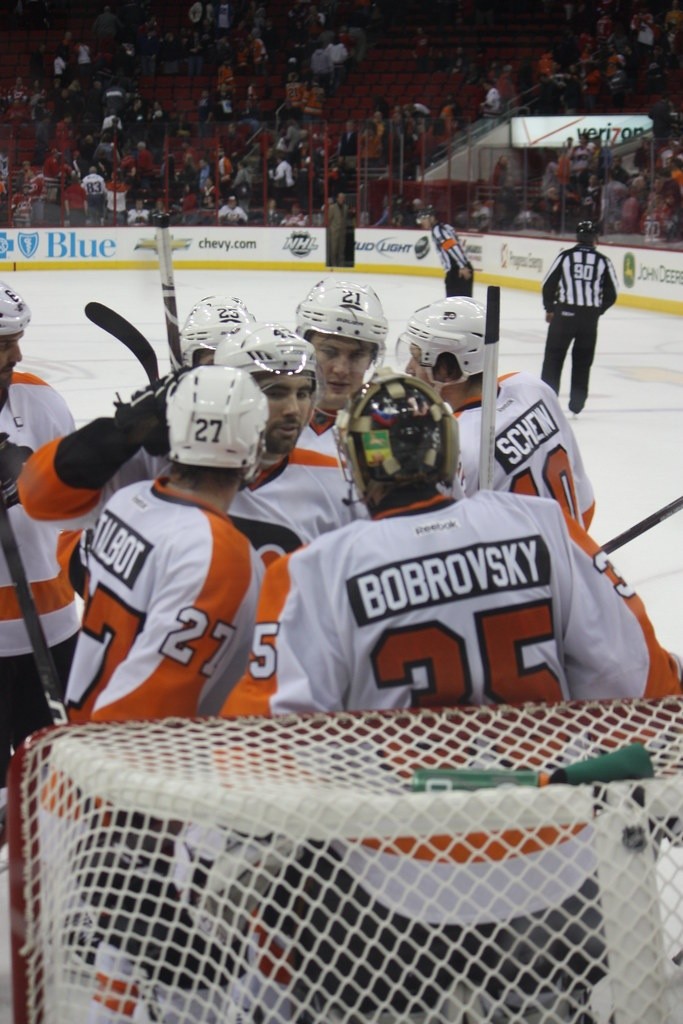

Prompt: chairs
[{"left": 0, "top": 0, "right": 683, "bottom": 242}]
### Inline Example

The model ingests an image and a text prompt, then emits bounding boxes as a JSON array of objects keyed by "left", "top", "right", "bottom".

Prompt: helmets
[
  {"left": 214, "top": 321, "right": 318, "bottom": 376},
  {"left": 1, "top": 280, "right": 31, "bottom": 336},
  {"left": 180, "top": 296, "right": 257, "bottom": 369},
  {"left": 334, "top": 366, "right": 461, "bottom": 494},
  {"left": 295, "top": 276, "right": 389, "bottom": 367},
  {"left": 576, "top": 219, "right": 601, "bottom": 243},
  {"left": 165, "top": 365, "right": 271, "bottom": 470},
  {"left": 398, "top": 296, "right": 487, "bottom": 384},
  {"left": 416, "top": 204, "right": 438, "bottom": 218}
]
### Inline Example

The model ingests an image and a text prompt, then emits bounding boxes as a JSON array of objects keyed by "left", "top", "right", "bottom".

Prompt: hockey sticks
[
  {"left": 474, "top": 286, "right": 501, "bottom": 492},
  {"left": 0, "top": 494, "right": 69, "bottom": 727},
  {"left": 600, "top": 496, "right": 682, "bottom": 555},
  {"left": 153, "top": 214, "right": 183, "bottom": 372},
  {"left": 87, "top": 302, "right": 160, "bottom": 385}
]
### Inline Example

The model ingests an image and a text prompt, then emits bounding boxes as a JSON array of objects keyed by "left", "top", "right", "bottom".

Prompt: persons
[
  {"left": 0, "top": 0, "right": 683, "bottom": 248},
  {"left": 0, "top": 280, "right": 112, "bottom": 884},
  {"left": 15, "top": 293, "right": 273, "bottom": 548},
  {"left": 387, "top": 297, "right": 596, "bottom": 543},
  {"left": 282, "top": 272, "right": 396, "bottom": 518},
  {"left": 29, "top": 367, "right": 296, "bottom": 1011},
  {"left": 175, "top": 359, "right": 683, "bottom": 1024},
  {"left": 323, "top": 192, "right": 354, "bottom": 267},
  {"left": 415, "top": 204, "right": 475, "bottom": 301},
  {"left": 167, "top": 324, "right": 371, "bottom": 720},
  {"left": 537, "top": 218, "right": 621, "bottom": 424}
]
